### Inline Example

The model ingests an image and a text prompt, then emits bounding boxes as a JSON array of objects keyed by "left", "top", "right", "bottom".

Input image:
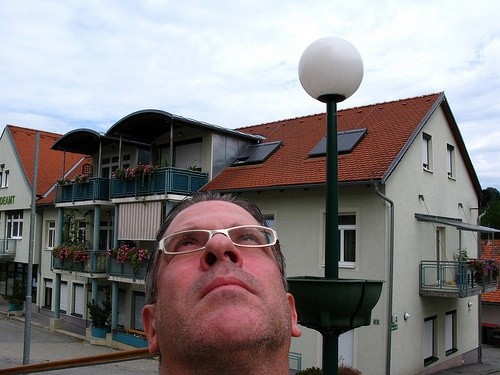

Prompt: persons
[{"left": 141, "top": 191, "right": 301, "bottom": 375}]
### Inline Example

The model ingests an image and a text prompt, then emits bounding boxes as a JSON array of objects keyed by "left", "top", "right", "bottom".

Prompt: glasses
[{"left": 151, "top": 225, "right": 285, "bottom": 303}]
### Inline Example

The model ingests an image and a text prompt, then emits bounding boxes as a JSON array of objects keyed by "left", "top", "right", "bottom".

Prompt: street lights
[{"left": 297, "top": 36, "right": 365, "bottom": 375}]
